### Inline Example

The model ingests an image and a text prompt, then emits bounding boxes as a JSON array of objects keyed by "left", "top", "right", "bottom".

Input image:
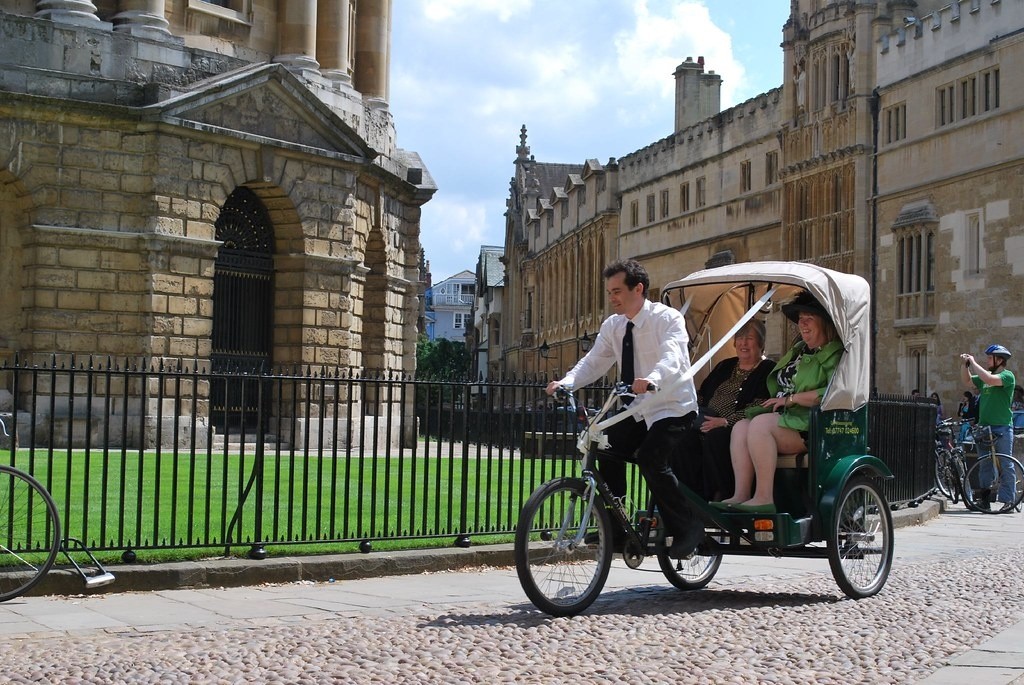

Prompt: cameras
[{"left": 962, "top": 353, "right": 968, "bottom": 359}]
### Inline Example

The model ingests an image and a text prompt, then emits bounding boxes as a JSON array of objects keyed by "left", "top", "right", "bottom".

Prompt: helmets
[{"left": 985, "top": 344, "right": 1011, "bottom": 361}]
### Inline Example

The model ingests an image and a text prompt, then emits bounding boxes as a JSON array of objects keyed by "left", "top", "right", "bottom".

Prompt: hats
[{"left": 782, "top": 289, "right": 834, "bottom": 325}]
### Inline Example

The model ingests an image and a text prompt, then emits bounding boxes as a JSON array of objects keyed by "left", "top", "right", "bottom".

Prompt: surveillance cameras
[{"left": 903, "top": 16, "right": 916, "bottom": 23}]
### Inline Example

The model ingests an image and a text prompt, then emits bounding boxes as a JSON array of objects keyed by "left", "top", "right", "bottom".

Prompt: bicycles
[
  {"left": 963, "top": 425, "right": 1024, "bottom": 515},
  {"left": 935, "top": 416, "right": 973, "bottom": 514},
  {"left": 1, "top": 465, "right": 63, "bottom": 601}
]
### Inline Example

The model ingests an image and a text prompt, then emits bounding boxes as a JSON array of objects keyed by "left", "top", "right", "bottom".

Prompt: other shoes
[
  {"left": 999, "top": 502, "right": 1014, "bottom": 513},
  {"left": 970, "top": 502, "right": 991, "bottom": 511},
  {"left": 584, "top": 524, "right": 625, "bottom": 545},
  {"left": 669, "top": 517, "right": 704, "bottom": 560},
  {"left": 708, "top": 501, "right": 730, "bottom": 512},
  {"left": 726, "top": 500, "right": 777, "bottom": 513}
]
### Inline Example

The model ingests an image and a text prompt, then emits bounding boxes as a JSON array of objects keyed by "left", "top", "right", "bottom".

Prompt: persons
[
  {"left": 931, "top": 392, "right": 942, "bottom": 425},
  {"left": 912, "top": 390, "right": 920, "bottom": 397},
  {"left": 958, "top": 385, "right": 1024, "bottom": 443},
  {"left": 548, "top": 259, "right": 703, "bottom": 560},
  {"left": 959, "top": 344, "right": 1016, "bottom": 514},
  {"left": 710, "top": 290, "right": 844, "bottom": 514},
  {"left": 672, "top": 318, "right": 777, "bottom": 501}
]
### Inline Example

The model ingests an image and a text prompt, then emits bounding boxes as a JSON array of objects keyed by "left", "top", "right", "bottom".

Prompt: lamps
[
  {"left": 540, "top": 339, "right": 558, "bottom": 369},
  {"left": 580, "top": 330, "right": 591, "bottom": 352}
]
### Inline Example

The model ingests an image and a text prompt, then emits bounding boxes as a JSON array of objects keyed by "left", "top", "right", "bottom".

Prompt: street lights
[
  {"left": 579, "top": 329, "right": 592, "bottom": 412},
  {"left": 538, "top": 338, "right": 551, "bottom": 432}
]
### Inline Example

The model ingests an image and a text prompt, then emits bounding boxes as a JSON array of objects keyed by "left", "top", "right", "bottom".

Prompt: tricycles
[{"left": 514, "top": 261, "right": 897, "bottom": 616}]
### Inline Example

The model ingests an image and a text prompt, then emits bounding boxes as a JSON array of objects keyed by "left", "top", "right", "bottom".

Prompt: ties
[
  {"left": 619, "top": 320, "right": 635, "bottom": 407},
  {"left": 976, "top": 396, "right": 978, "bottom": 400}
]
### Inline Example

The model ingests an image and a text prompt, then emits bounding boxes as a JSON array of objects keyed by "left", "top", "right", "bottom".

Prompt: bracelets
[
  {"left": 790, "top": 394, "right": 793, "bottom": 401},
  {"left": 725, "top": 420, "right": 728, "bottom": 427}
]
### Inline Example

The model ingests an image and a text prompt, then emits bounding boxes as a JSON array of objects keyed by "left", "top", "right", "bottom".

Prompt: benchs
[{"left": 777, "top": 405, "right": 816, "bottom": 468}]
